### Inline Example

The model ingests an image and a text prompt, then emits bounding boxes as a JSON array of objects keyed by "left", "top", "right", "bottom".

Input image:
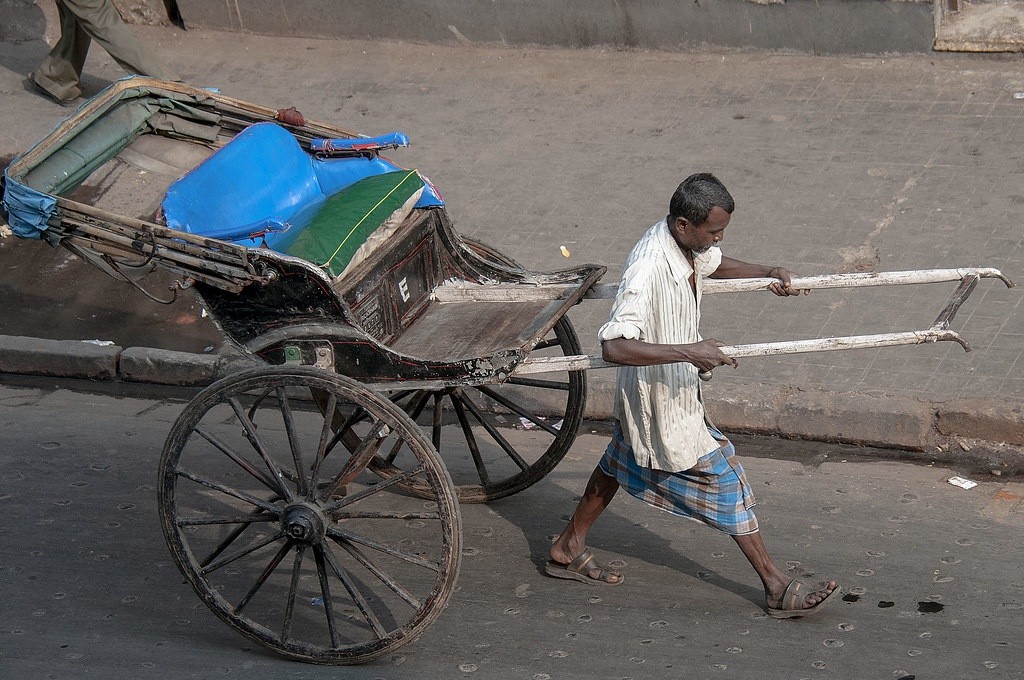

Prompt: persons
[
  {"left": 27, "top": 0, "right": 185, "bottom": 108},
  {"left": 541, "top": 172, "right": 842, "bottom": 618}
]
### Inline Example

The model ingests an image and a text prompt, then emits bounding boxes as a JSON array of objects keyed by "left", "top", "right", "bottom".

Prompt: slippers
[
  {"left": 544, "top": 549, "right": 624, "bottom": 587},
  {"left": 768, "top": 578, "right": 841, "bottom": 618}
]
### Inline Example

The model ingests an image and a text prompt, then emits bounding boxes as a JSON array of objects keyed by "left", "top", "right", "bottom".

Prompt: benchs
[{"left": 154, "top": 120, "right": 445, "bottom": 348}]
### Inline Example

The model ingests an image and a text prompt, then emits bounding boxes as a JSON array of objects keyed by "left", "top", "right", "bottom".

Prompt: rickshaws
[{"left": 1, "top": 73, "right": 1013, "bottom": 667}]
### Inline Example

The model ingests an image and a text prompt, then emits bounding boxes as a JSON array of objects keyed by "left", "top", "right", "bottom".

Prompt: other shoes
[{"left": 27, "top": 71, "right": 78, "bottom": 107}]
